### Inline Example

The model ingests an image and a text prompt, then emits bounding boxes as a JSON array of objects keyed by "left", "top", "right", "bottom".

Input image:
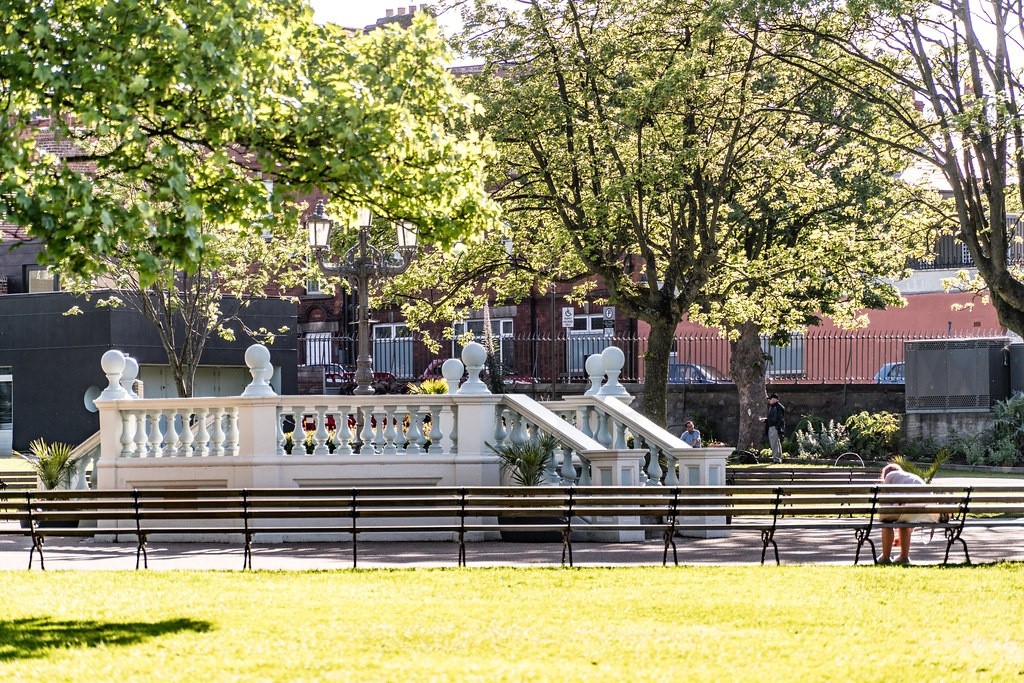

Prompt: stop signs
[{"left": 603, "top": 305, "right": 616, "bottom": 321}]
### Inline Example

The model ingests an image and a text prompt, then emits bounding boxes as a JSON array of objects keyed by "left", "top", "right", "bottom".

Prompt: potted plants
[
  {"left": 11, "top": 437, "right": 80, "bottom": 536},
  {"left": 484, "top": 430, "right": 568, "bottom": 542}
]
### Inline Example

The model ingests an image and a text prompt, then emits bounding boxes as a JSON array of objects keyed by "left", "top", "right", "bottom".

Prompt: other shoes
[
  {"left": 774, "top": 462, "right": 781, "bottom": 464},
  {"left": 893, "top": 557, "right": 910, "bottom": 564},
  {"left": 876, "top": 558, "right": 891, "bottom": 564}
]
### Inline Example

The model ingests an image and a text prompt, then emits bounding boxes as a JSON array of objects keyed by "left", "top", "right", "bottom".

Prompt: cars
[
  {"left": 663, "top": 363, "right": 735, "bottom": 383},
  {"left": 417, "top": 357, "right": 539, "bottom": 384},
  {"left": 298, "top": 363, "right": 397, "bottom": 380}
]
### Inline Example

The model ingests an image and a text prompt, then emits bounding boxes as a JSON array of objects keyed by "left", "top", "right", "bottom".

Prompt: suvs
[{"left": 870, "top": 360, "right": 907, "bottom": 384}]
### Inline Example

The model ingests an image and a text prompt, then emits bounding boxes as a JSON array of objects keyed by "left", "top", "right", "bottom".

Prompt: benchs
[
  {"left": 0, "top": 471, "right": 93, "bottom": 522},
  {"left": 0, "top": 485, "right": 1024, "bottom": 572},
  {"left": 645, "top": 468, "right": 882, "bottom": 518}
]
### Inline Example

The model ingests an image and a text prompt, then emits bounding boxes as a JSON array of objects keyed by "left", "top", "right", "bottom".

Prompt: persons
[
  {"left": 874, "top": 463, "right": 941, "bottom": 566},
  {"left": 679, "top": 421, "right": 702, "bottom": 449},
  {"left": 759, "top": 393, "right": 786, "bottom": 464}
]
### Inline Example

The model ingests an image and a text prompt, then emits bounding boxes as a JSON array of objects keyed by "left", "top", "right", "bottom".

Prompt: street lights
[{"left": 305, "top": 199, "right": 422, "bottom": 396}]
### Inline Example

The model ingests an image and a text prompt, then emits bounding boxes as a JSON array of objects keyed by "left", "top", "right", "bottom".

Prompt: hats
[{"left": 767, "top": 394, "right": 779, "bottom": 400}]
